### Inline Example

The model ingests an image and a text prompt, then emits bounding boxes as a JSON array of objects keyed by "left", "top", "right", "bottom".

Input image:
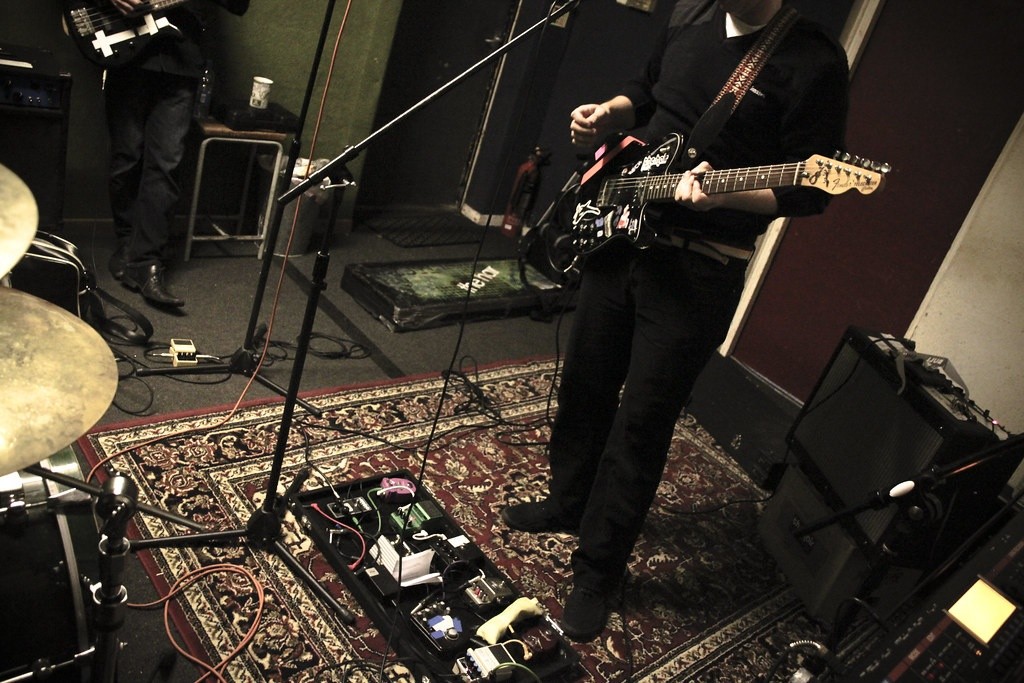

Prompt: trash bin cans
[{"left": 256, "top": 154, "right": 333, "bottom": 257}]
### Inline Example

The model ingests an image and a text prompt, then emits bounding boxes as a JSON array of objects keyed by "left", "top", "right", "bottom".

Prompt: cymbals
[
  {"left": 0, "top": 163, "right": 40, "bottom": 283},
  {"left": 0, "top": 284, "right": 120, "bottom": 479}
]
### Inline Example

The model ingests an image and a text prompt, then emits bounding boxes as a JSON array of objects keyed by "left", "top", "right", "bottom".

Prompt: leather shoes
[
  {"left": 109, "top": 248, "right": 129, "bottom": 280},
  {"left": 120, "top": 265, "right": 184, "bottom": 308}
]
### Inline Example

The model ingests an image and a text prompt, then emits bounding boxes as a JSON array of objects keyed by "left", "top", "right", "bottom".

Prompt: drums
[{"left": 0, "top": 440, "right": 109, "bottom": 683}]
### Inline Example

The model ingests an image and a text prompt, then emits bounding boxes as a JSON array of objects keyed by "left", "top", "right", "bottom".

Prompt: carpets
[{"left": 78, "top": 353, "right": 830, "bottom": 683}]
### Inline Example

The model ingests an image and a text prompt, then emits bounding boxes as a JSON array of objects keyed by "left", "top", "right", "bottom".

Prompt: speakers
[
  {"left": 0, "top": 43, "right": 72, "bottom": 239},
  {"left": 753, "top": 326, "right": 1024, "bottom": 634}
]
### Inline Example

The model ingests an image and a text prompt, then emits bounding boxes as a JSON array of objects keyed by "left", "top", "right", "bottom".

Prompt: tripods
[{"left": 129, "top": 0, "right": 580, "bottom": 625}]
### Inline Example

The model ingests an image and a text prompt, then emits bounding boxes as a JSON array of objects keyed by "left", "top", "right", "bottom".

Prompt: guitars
[
  {"left": 543, "top": 129, "right": 894, "bottom": 276},
  {"left": 62, "top": 0, "right": 188, "bottom": 72}
]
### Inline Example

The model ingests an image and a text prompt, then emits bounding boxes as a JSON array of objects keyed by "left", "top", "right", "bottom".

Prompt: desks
[{"left": 179, "top": 115, "right": 287, "bottom": 262}]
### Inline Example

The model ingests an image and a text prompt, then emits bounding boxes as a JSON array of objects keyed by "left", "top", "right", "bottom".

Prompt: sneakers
[
  {"left": 502, "top": 498, "right": 582, "bottom": 532},
  {"left": 562, "top": 585, "right": 604, "bottom": 640}
]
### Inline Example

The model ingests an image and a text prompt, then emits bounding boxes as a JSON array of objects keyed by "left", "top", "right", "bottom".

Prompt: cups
[{"left": 249, "top": 77, "right": 274, "bottom": 109}]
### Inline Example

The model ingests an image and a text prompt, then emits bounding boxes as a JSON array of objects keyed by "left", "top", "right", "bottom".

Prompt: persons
[
  {"left": 104, "top": 0, "right": 252, "bottom": 309},
  {"left": 502, "top": 0, "right": 850, "bottom": 642}
]
